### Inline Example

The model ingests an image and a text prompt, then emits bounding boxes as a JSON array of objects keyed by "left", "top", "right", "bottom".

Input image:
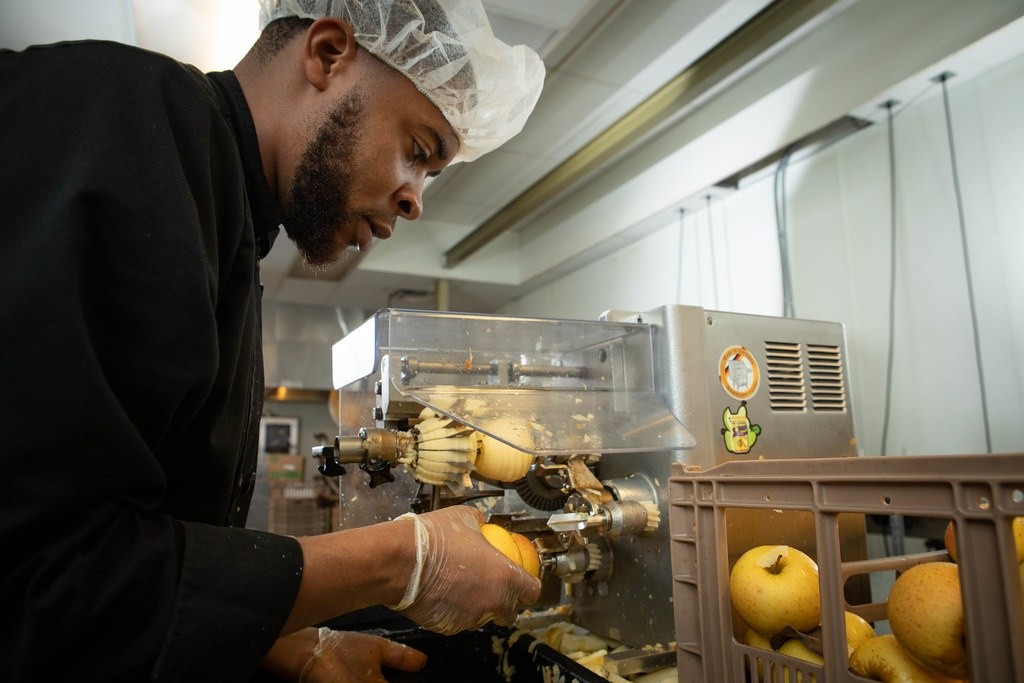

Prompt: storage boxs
[{"left": 666, "top": 452, "right": 1024, "bottom": 682}]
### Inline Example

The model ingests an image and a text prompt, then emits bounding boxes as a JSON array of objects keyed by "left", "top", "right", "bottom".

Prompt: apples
[
  {"left": 415, "top": 415, "right": 478, "bottom": 486},
  {"left": 473, "top": 418, "right": 534, "bottom": 481},
  {"left": 729, "top": 517, "right": 1024, "bottom": 683},
  {"left": 481, "top": 522, "right": 540, "bottom": 579},
  {"left": 513, "top": 605, "right": 634, "bottom": 679}
]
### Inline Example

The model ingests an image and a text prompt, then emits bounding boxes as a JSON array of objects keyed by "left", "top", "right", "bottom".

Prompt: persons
[{"left": 0, "top": 0, "right": 548, "bottom": 683}]
